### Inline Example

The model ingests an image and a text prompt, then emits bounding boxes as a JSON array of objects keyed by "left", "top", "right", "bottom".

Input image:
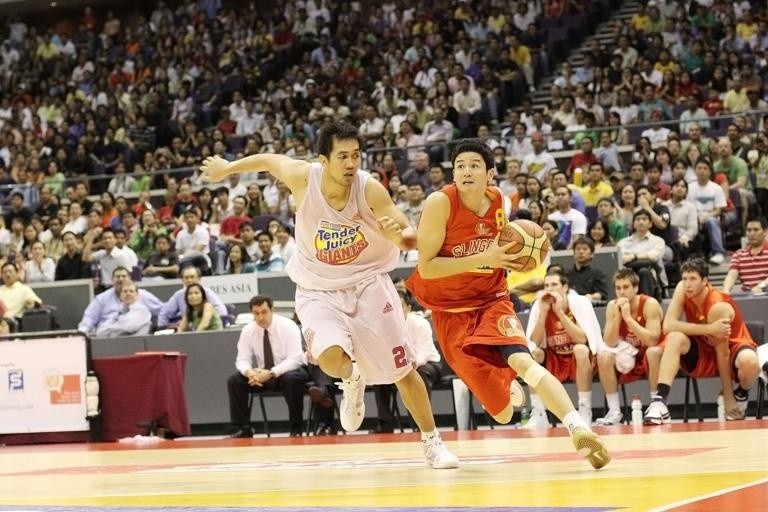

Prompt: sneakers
[
  {"left": 338, "top": 361, "right": 366, "bottom": 433},
  {"left": 596, "top": 408, "right": 625, "bottom": 424},
  {"left": 726, "top": 387, "right": 749, "bottom": 420},
  {"left": 509, "top": 377, "right": 526, "bottom": 408},
  {"left": 421, "top": 428, "right": 460, "bottom": 469},
  {"left": 643, "top": 396, "right": 671, "bottom": 424},
  {"left": 572, "top": 425, "right": 611, "bottom": 470},
  {"left": 577, "top": 407, "right": 592, "bottom": 426},
  {"left": 517, "top": 409, "right": 550, "bottom": 428},
  {"left": 709, "top": 253, "right": 726, "bottom": 265}
]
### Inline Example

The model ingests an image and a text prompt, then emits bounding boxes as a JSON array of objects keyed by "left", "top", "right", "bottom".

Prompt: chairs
[{"left": 0, "top": 1, "right": 768, "bottom": 443}]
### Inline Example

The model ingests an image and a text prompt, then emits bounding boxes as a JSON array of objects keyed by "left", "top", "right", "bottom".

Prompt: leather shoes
[
  {"left": 231, "top": 428, "right": 252, "bottom": 438},
  {"left": 308, "top": 386, "right": 333, "bottom": 405}
]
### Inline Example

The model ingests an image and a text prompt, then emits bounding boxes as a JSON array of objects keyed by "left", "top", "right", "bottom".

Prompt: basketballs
[{"left": 499, "top": 219, "right": 550, "bottom": 273}]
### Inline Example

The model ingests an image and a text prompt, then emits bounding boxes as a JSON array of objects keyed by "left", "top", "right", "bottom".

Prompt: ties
[{"left": 263, "top": 328, "right": 274, "bottom": 369}]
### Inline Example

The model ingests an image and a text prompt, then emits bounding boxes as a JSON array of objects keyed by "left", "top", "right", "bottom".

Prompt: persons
[
  {"left": 227, "top": 295, "right": 310, "bottom": 438},
  {"left": 596, "top": 269, "right": 664, "bottom": 426},
  {"left": 518, "top": 270, "right": 603, "bottom": 429},
  {"left": 303, "top": 348, "right": 343, "bottom": 436},
  {"left": 644, "top": 257, "right": 761, "bottom": 425},
  {"left": 200, "top": 122, "right": 462, "bottom": 470},
  {"left": 404, "top": 139, "right": 612, "bottom": 469},
  {"left": 0, "top": 0, "right": 768, "bottom": 338},
  {"left": 369, "top": 291, "right": 443, "bottom": 434}
]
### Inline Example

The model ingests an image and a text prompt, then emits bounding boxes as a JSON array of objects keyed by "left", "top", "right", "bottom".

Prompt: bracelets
[{"left": 269, "top": 369, "right": 275, "bottom": 379}]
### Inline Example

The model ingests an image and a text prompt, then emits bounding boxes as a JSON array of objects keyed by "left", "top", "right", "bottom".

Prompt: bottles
[
  {"left": 85, "top": 371, "right": 102, "bottom": 418},
  {"left": 520, "top": 404, "right": 529, "bottom": 426},
  {"left": 631, "top": 393, "right": 643, "bottom": 425},
  {"left": 718, "top": 390, "right": 724, "bottom": 420}
]
